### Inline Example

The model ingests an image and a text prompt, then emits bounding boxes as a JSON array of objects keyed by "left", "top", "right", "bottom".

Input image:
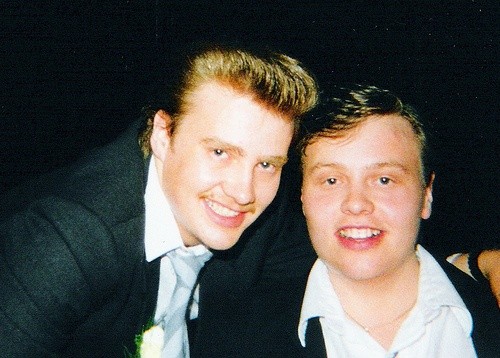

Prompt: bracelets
[{"left": 469, "top": 249, "right": 488, "bottom": 280}]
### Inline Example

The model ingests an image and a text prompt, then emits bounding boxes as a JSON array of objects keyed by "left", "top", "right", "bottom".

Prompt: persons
[
  {"left": 0, "top": 49, "right": 318, "bottom": 358},
  {"left": 205, "top": 83, "right": 500, "bottom": 358}
]
